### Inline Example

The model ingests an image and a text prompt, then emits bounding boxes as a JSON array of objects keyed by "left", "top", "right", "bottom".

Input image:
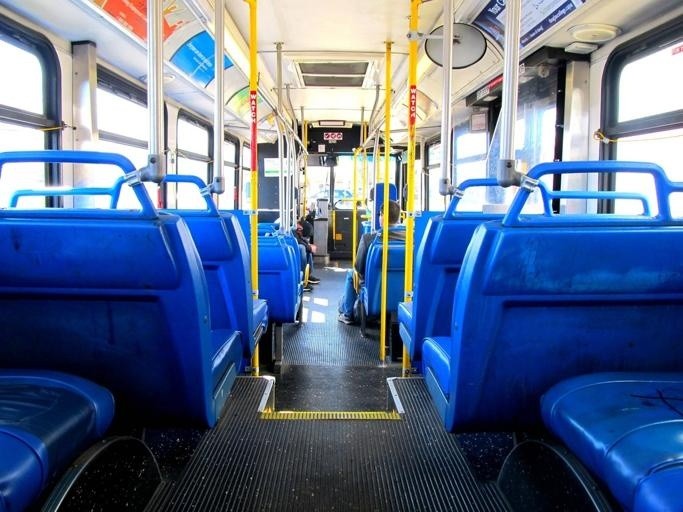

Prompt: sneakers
[
  {"left": 303, "top": 284, "right": 313, "bottom": 291},
  {"left": 339, "top": 313, "right": 353, "bottom": 324},
  {"left": 308, "top": 275, "right": 320, "bottom": 283}
]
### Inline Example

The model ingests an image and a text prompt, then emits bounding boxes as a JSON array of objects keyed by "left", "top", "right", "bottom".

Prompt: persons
[
  {"left": 337, "top": 199, "right": 405, "bottom": 326},
  {"left": 291, "top": 220, "right": 320, "bottom": 292}
]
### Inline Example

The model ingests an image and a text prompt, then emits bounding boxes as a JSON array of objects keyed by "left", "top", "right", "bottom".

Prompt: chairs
[
  {"left": 356, "top": 161, "right": 683, "bottom": 512},
  {"left": 0, "top": 150, "right": 302, "bottom": 510}
]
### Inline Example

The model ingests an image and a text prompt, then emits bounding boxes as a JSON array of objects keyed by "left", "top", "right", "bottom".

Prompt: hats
[{"left": 301, "top": 218, "right": 313, "bottom": 237}]
